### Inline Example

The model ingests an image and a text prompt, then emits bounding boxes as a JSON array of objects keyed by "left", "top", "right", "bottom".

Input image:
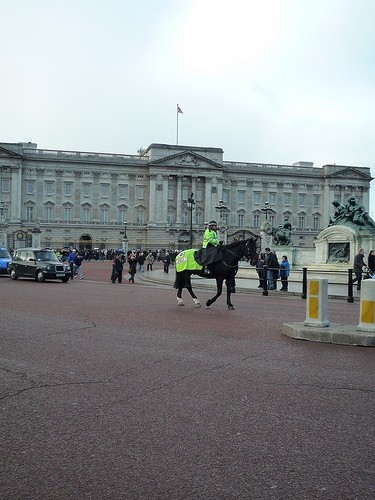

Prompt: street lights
[
  {"left": 215, "top": 199, "right": 227, "bottom": 219},
  {"left": 261, "top": 202, "right": 272, "bottom": 221},
  {"left": 188, "top": 192, "right": 196, "bottom": 248},
  {"left": 124, "top": 222, "right": 127, "bottom": 237}
]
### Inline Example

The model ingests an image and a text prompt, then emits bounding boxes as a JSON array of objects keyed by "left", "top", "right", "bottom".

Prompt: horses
[{"left": 173, "top": 237, "right": 259, "bottom": 310}]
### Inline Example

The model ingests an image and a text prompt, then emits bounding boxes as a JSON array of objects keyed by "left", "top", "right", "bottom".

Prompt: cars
[{"left": 0, "top": 247, "right": 71, "bottom": 282}]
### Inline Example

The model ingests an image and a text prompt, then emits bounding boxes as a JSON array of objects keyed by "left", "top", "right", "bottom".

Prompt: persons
[
  {"left": 352, "top": 249, "right": 367, "bottom": 292},
  {"left": 201, "top": 220, "right": 223, "bottom": 276},
  {"left": 367, "top": 249, "right": 375, "bottom": 278},
  {"left": 51, "top": 241, "right": 289, "bottom": 292}
]
